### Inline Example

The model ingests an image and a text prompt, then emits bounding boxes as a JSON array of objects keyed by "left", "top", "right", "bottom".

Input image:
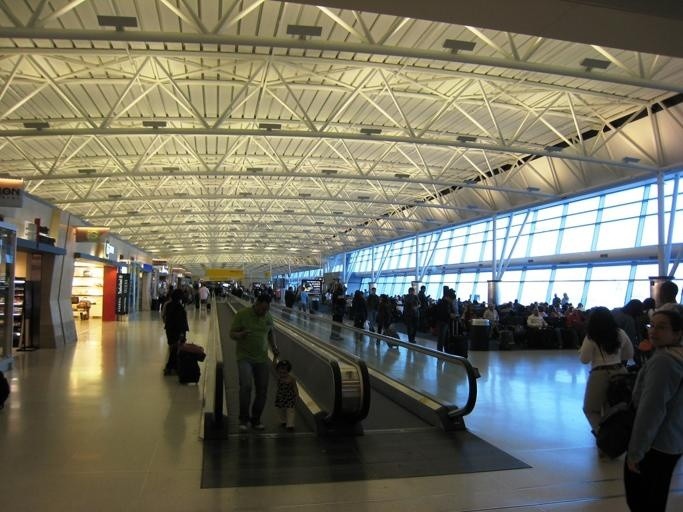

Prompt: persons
[
  {"left": 244, "top": 283, "right": 310, "bottom": 320},
  {"left": 271, "top": 349, "right": 299, "bottom": 431},
  {"left": 229, "top": 293, "right": 281, "bottom": 431},
  {"left": 156, "top": 278, "right": 244, "bottom": 376},
  {"left": 578, "top": 282, "right": 682, "bottom": 512},
  {"left": 322, "top": 282, "right": 589, "bottom": 359}
]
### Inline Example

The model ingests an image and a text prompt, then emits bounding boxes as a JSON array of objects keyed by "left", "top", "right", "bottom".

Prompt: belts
[{"left": 592, "top": 363, "right": 624, "bottom": 371}]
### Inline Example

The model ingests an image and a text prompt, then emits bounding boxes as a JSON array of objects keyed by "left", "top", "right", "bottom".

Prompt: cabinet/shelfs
[{"left": 0, "top": 277, "right": 26, "bottom": 345}]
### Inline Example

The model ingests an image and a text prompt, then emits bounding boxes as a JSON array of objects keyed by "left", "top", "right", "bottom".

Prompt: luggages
[
  {"left": 444, "top": 336, "right": 469, "bottom": 357},
  {"left": 178, "top": 354, "right": 200, "bottom": 383}
]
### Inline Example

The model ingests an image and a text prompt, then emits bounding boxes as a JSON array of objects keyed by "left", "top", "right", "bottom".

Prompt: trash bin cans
[{"left": 468, "top": 319, "right": 490, "bottom": 351}]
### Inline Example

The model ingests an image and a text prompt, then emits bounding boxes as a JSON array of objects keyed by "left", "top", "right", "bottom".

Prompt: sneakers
[
  {"left": 239, "top": 423, "right": 265, "bottom": 432},
  {"left": 281, "top": 420, "right": 296, "bottom": 429}
]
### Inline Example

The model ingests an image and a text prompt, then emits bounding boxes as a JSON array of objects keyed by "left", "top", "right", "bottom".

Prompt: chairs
[{"left": 425, "top": 296, "right": 593, "bottom": 348}]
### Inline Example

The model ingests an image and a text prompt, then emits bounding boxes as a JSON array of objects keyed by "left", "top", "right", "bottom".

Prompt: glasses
[{"left": 645, "top": 323, "right": 675, "bottom": 331}]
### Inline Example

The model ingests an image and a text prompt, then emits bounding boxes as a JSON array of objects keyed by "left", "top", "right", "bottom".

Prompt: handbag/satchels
[
  {"left": 178, "top": 342, "right": 206, "bottom": 362},
  {"left": 606, "top": 372, "right": 635, "bottom": 405},
  {"left": 595, "top": 403, "right": 634, "bottom": 459}
]
